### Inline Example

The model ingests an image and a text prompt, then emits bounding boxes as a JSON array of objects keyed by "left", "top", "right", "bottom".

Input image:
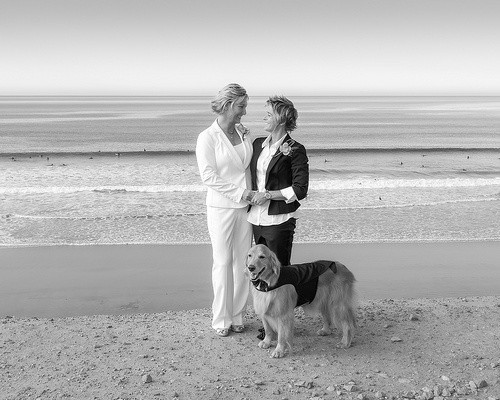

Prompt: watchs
[{"left": 263, "top": 190, "right": 272, "bottom": 200}]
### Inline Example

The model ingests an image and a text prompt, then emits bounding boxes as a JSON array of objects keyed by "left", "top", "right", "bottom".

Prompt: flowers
[
  {"left": 239, "top": 122, "right": 252, "bottom": 134},
  {"left": 280, "top": 141, "right": 294, "bottom": 158}
]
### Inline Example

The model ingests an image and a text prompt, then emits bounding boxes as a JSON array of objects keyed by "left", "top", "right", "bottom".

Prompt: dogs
[{"left": 245, "top": 244, "right": 358, "bottom": 359}]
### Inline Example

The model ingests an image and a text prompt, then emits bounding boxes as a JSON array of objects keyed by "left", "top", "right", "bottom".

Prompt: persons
[
  {"left": 249, "top": 97, "right": 310, "bottom": 341},
  {"left": 196, "top": 84, "right": 262, "bottom": 336}
]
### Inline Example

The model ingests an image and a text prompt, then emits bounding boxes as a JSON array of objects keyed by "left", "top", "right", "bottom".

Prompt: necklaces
[{"left": 223, "top": 128, "right": 235, "bottom": 135}]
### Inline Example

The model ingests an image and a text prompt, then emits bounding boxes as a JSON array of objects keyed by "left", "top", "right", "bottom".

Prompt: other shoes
[
  {"left": 232, "top": 325, "right": 244, "bottom": 332},
  {"left": 216, "top": 329, "right": 230, "bottom": 337},
  {"left": 257, "top": 327, "right": 264, "bottom": 332},
  {"left": 256, "top": 332, "right": 266, "bottom": 340}
]
